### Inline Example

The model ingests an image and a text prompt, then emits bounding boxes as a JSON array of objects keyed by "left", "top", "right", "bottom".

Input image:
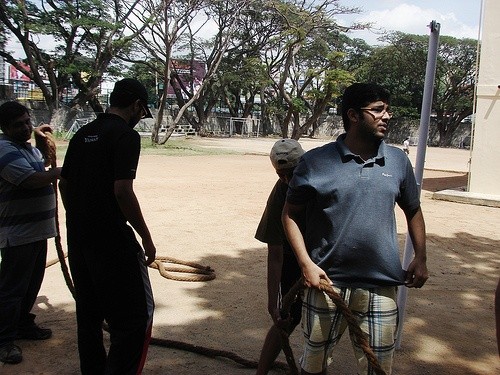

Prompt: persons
[
  {"left": 403, "top": 138, "right": 409, "bottom": 156},
  {"left": 281, "top": 82, "right": 429, "bottom": 375},
  {"left": 0, "top": 101, "right": 63, "bottom": 365},
  {"left": 255, "top": 138, "right": 308, "bottom": 375},
  {"left": 59, "top": 78, "right": 156, "bottom": 375}
]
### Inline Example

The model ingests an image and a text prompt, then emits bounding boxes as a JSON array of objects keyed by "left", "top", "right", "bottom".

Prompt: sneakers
[
  {"left": 15, "top": 324, "right": 52, "bottom": 339},
  {"left": 0, "top": 342, "right": 23, "bottom": 363}
]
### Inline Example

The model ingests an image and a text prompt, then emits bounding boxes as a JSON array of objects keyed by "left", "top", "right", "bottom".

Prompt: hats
[
  {"left": 114, "top": 78, "right": 154, "bottom": 119},
  {"left": 270, "top": 138, "right": 304, "bottom": 169}
]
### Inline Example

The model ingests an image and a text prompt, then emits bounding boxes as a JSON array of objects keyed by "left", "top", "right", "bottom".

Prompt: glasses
[
  {"left": 351, "top": 107, "right": 393, "bottom": 119},
  {"left": 137, "top": 100, "right": 147, "bottom": 118}
]
